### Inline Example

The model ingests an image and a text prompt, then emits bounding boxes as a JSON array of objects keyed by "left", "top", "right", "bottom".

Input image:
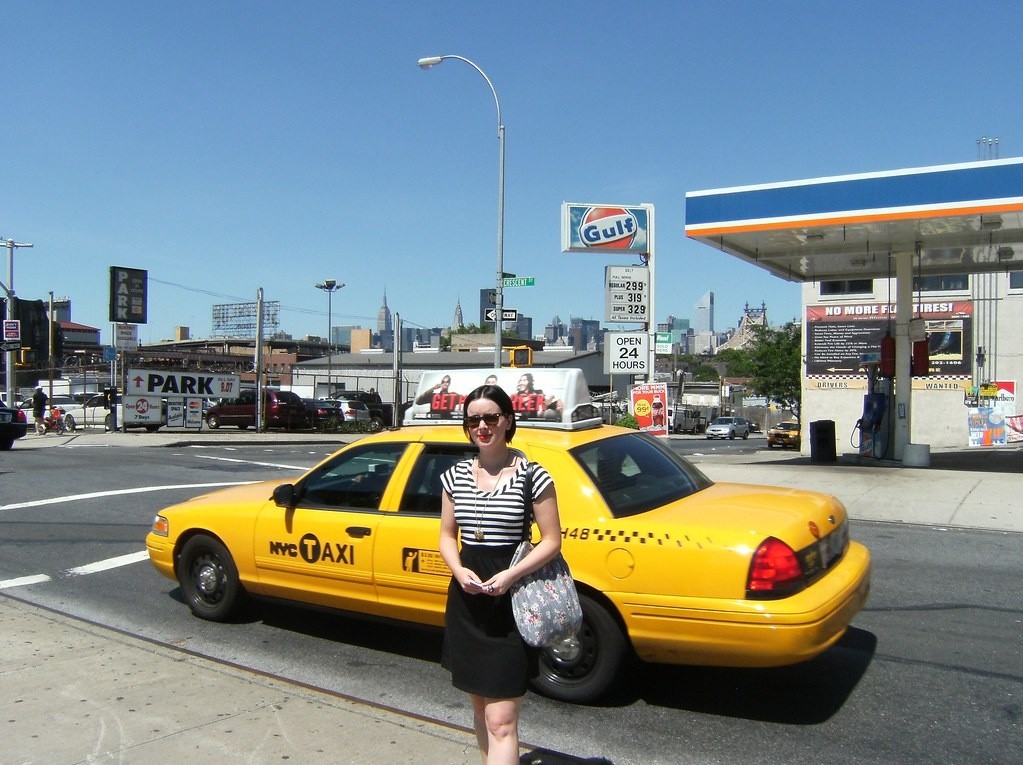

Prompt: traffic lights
[{"left": 509, "top": 345, "right": 532, "bottom": 367}]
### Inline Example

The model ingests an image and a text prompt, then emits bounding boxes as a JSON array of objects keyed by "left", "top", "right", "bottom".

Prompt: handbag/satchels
[{"left": 507, "top": 461, "right": 584, "bottom": 647}]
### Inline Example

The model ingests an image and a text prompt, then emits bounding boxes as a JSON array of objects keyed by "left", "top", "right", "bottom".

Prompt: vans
[
  {"left": 706, "top": 417, "right": 749, "bottom": 440},
  {"left": 591, "top": 402, "right": 699, "bottom": 433}
]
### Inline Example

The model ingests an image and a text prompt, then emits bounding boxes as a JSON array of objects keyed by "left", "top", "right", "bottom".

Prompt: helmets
[{"left": 35, "top": 386, "right": 43, "bottom": 391}]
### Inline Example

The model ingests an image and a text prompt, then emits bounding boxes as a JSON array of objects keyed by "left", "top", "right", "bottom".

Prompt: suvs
[{"left": 203, "top": 388, "right": 306, "bottom": 429}]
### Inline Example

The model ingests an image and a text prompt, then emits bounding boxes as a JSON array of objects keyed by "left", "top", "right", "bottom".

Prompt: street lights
[
  {"left": 417, "top": 54, "right": 505, "bottom": 368},
  {"left": 315, "top": 281, "right": 345, "bottom": 398}
]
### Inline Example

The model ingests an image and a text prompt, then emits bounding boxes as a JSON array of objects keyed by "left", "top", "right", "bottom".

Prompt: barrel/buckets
[{"left": 810, "top": 420, "right": 837, "bottom": 463}]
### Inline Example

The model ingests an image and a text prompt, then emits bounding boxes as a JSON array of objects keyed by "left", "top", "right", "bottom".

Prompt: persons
[
  {"left": 416, "top": 375, "right": 458, "bottom": 420},
  {"left": 352, "top": 475, "right": 386, "bottom": 510},
  {"left": 31, "top": 386, "right": 47, "bottom": 436},
  {"left": 516, "top": 373, "right": 542, "bottom": 418},
  {"left": 485, "top": 375, "right": 497, "bottom": 385},
  {"left": 439, "top": 385, "right": 560, "bottom": 765}
]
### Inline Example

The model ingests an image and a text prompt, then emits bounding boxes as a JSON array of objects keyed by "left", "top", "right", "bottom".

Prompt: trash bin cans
[{"left": 809, "top": 420, "right": 837, "bottom": 462}]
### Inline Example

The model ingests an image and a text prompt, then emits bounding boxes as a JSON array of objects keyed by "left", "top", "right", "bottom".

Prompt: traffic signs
[
  {"left": 484, "top": 277, "right": 535, "bottom": 322},
  {"left": 0, "top": 341, "right": 21, "bottom": 353}
]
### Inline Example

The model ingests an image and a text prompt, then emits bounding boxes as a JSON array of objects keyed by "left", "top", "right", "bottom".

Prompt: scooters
[{"left": 38, "top": 406, "right": 64, "bottom": 435}]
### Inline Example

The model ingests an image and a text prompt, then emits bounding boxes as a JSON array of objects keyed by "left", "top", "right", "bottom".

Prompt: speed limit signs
[{"left": 604, "top": 332, "right": 650, "bottom": 374}]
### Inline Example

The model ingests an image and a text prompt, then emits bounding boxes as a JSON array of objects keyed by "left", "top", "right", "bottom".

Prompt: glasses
[{"left": 465, "top": 413, "right": 505, "bottom": 429}]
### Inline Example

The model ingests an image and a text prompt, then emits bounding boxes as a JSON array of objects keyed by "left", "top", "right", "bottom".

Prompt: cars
[
  {"left": 19, "top": 396, "right": 89, "bottom": 424},
  {"left": 322, "top": 400, "right": 371, "bottom": 424},
  {"left": 332, "top": 391, "right": 413, "bottom": 432},
  {"left": 0, "top": 400, "right": 27, "bottom": 450},
  {"left": 144, "top": 367, "right": 873, "bottom": 704},
  {"left": 0, "top": 392, "right": 26, "bottom": 407},
  {"left": 767, "top": 422, "right": 800, "bottom": 448},
  {"left": 746, "top": 420, "right": 759, "bottom": 432},
  {"left": 300, "top": 398, "right": 345, "bottom": 428},
  {"left": 63, "top": 393, "right": 167, "bottom": 432}
]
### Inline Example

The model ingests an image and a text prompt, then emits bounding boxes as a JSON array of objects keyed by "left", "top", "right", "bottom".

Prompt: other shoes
[
  {"left": 34, "top": 432, "right": 40, "bottom": 435},
  {"left": 43, "top": 427, "right": 48, "bottom": 435}
]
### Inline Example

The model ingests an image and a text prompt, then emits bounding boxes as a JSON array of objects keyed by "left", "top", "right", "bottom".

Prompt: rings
[{"left": 486, "top": 586, "right": 494, "bottom": 593}]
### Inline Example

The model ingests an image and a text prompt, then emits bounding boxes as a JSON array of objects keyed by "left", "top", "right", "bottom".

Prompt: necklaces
[{"left": 473, "top": 450, "right": 510, "bottom": 539}]
